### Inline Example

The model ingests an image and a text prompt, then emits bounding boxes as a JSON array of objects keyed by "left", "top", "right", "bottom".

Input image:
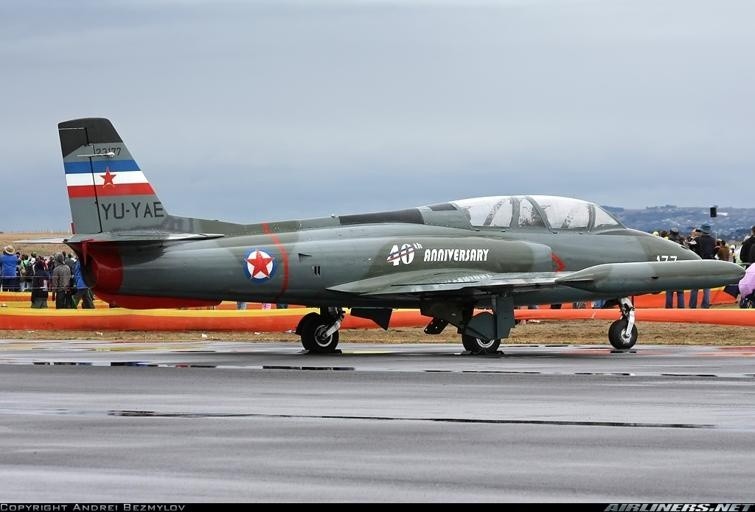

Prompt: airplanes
[{"left": 12, "top": 117, "right": 747, "bottom": 355}]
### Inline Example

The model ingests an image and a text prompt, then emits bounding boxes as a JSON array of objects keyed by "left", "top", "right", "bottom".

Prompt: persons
[
  {"left": 651, "top": 223, "right": 755, "bottom": 308},
  {"left": 527, "top": 299, "right": 586, "bottom": 310},
  {"left": 179, "top": 302, "right": 289, "bottom": 310},
  {"left": 0, "top": 245, "right": 95, "bottom": 309}
]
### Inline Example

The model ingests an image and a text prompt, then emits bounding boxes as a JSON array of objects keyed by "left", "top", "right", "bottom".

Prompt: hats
[
  {"left": 668, "top": 228, "right": 681, "bottom": 240},
  {"left": 696, "top": 222, "right": 712, "bottom": 234},
  {"left": 4, "top": 245, "right": 15, "bottom": 255}
]
